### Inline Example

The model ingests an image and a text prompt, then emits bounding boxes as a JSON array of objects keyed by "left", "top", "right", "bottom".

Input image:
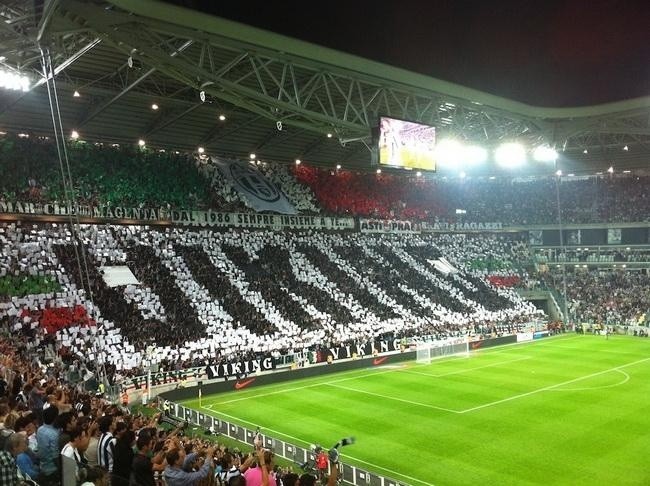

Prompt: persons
[
  {"left": 0, "top": 127, "right": 650, "bottom": 388},
  {"left": 1, "top": 358, "right": 337, "bottom": 485}
]
